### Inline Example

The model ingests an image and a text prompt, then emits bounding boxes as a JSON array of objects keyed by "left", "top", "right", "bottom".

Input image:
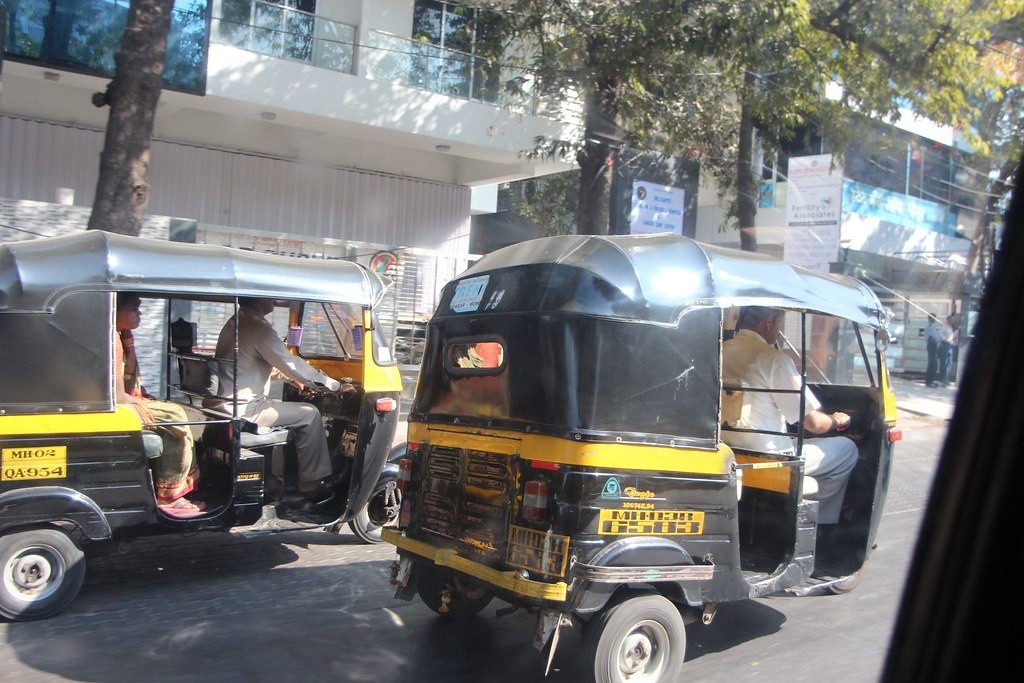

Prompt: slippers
[{"left": 159, "top": 498, "right": 207, "bottom": 510}]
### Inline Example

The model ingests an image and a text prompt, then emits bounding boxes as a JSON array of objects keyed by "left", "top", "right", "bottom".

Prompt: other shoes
[
  {"left": 296, "top": 484, "right": 347, "bottom": 513},
  {"left": 817, "top": 524, "right": 849, "bottom": 540}
]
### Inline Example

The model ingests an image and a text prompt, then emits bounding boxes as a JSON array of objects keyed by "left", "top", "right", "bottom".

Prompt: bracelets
[{"left": 829, "top": 414, "right": 839, "bottom": 432}]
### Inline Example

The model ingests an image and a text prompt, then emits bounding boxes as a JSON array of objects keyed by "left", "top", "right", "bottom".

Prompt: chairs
[
  {"left": 177, "top": 353, "right": 290, "bottom": 448},
  {"left": 722, "top": 377, "right": 819, "bottom": 495}
]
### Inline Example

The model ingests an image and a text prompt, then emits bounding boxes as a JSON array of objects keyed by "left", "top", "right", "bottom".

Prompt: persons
[
  {"left": 214, "top": 296, "right": 356, "bottom": 497},
  {"left": 115, "top": 291, "right": 207, "bottom": 513},
  {"left": 721, "top": 305, "right": 858, "bottom": 551},
  {"left": 925, "top": 313, "right": 956, "bottom": 387}
]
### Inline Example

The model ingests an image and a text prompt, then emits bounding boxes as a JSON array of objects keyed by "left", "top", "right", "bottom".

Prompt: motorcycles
[
  {"left": 380, "top": 231, "right": 903, "bottom": 683},
  {"left": 0, "top": 229, "right": 405, "bottom": 623}
]
